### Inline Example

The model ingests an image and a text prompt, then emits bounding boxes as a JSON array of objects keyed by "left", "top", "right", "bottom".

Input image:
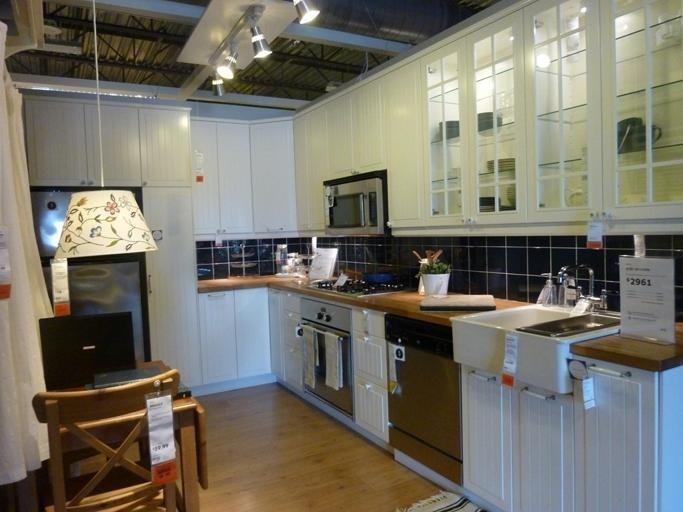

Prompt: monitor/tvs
[{"left": 39, "top": 311, "right": 137, "bottom": 391}]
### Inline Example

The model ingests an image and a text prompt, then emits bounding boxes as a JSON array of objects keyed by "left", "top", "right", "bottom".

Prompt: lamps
[
  {"left": 241, "top": 4, "right": 272, "bottom": 59},
  {"left": 291, "top": 0, "right": 321, "bottom": 24},
  {"left": 217, "top": 35, "right": 239, "bottom": 80},
  {"left": 54, "top": 0, "right": 159, "bottom": 258}
]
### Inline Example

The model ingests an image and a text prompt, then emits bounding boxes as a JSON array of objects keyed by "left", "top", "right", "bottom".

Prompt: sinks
[
  {"left": 516, "top": 310, "right": 621, "bottom": 339},
  {"left": 448, "top": 303, "right": 583, "bottom": 331}
]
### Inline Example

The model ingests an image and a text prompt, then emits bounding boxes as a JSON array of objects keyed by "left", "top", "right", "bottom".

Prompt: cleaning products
[
  {"left": 557, "top": 272, "right": 571, "bottom": 307},
  {"left": 540, "top": 273, "right": 559, "bottom": 307},
  {"left": 542, "top": 272, "right": 557, "bottom": 306}
]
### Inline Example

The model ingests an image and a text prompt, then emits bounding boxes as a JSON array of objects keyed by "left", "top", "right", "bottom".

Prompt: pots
[{"left": 363, "top": 270, "right": 397, "bottom": 283}]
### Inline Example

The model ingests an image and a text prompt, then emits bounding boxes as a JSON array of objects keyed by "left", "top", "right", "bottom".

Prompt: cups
[{"left": 617, "top": 116, "right": 663, "bottom": 153}]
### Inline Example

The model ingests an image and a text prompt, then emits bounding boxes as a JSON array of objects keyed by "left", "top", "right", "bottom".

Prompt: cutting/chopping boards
[{"left": 420, "top": 294, "right": 496, "bottom": 312}]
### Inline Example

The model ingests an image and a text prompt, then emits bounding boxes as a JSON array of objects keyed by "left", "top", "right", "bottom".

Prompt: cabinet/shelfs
[
  {"left": 461, "top": 362, "right": 573, "bottom": 512},
  {"left": 422, "top": 0, "right": 526, "bottom": 240},
  {"left": 141, "top": 187, "right": 203, "bottom": 400},
  {"left": 192, "top": 109, "right": 294, "bottom": 240},
  {"left": 570, "top": 345, "right": 682, "bottom": 512},
  {"left": 138, "top": 106, "right": 193, "bottom": 187},
  {"left": 268, "top": 281, "right": 390, "bottom": 459},
  {"left": 526, "top": 1, "right": 683, "bottom": 237},
  {"left": 199, "top": 277, "right": 276, "bottom": 395},
  {"left": 294, "top": 44, "right": 422, "bottom": 234},
  {"left": 22, "top": 94, "right": 141, "bottom": 188}
]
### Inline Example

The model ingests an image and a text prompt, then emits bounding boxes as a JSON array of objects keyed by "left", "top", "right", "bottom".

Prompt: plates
[
  {"left": 480, "top": 196, "right": 501, "bottom": 212},
  {"left": 506, "top": 186, "right": 516, "bottom": 207},
  {"left": 439, "top": 121, "right": 459, "bottom": 138},
  {"left": 478, "top": 112, "right": 502, "bottom": 131},
  {"left": 487, "top": 159, "right": 515, "bottom": 172}
]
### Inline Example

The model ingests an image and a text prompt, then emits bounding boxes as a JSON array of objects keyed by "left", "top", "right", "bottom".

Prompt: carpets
[{"left": 401, "top": 491, "right": 486, "bottom": 512}]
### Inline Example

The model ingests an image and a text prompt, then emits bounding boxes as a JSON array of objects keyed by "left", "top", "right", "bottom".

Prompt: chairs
[{"left": 32, "top": 369, "right": 182, "bottom": 512}]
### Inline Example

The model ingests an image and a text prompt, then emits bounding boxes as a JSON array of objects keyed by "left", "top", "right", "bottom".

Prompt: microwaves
[{"left": 324, "top": 170, "right": 388, "bottom": 237}]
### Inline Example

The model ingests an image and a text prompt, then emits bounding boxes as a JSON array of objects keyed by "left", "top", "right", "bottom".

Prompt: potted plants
[{"left": 417, "top": 264, "right": 452, "bottom": 296}]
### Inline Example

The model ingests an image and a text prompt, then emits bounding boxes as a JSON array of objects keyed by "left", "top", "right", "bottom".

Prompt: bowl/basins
[{"left": 422, "top": 274, "right": 449, "bottom": 296}]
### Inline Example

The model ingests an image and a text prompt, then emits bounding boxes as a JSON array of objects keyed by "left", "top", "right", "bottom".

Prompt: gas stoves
[{"left": 317, "top": 278, "right": 409, "bottom": 297}]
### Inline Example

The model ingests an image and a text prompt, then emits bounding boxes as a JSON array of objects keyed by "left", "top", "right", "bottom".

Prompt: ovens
[{"left": 298, "top": 297, "right": 356, "bottom": 419}]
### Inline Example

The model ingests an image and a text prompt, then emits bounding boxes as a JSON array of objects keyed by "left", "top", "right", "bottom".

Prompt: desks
[{"left": 41, "top": 358, "right": 207, "bottom": 510}]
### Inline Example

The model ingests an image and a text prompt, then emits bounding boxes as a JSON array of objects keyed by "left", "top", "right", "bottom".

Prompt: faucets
[{"left": 558, "top": 263, "right": 608, "bottom": 313}]
